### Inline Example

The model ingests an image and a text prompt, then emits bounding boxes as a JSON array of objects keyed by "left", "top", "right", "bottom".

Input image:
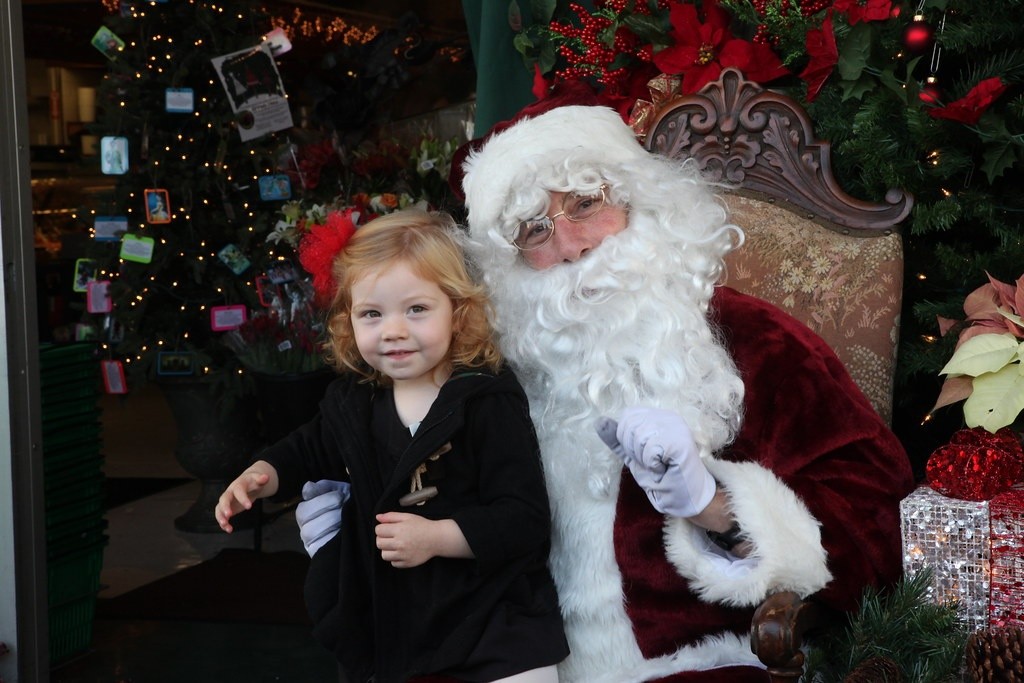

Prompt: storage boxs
[{"left": 900, "top": 483, "right": 1024, "bottom": 631}]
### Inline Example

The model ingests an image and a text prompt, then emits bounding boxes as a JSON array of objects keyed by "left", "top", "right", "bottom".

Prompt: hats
[{"left": 450, "top": 86, "right": 651, "bottom": 265}]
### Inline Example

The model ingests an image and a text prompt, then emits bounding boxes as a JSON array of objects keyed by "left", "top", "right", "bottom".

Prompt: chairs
[{"left": 647, "top": 68, "right": 915, "bottom": 682}]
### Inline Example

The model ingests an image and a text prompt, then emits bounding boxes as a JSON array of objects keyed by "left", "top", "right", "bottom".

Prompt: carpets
[{"left": 100, "top": 546, "right": 309, "bottom": 626}]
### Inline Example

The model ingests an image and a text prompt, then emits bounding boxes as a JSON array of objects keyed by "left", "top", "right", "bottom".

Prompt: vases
[{"left": 246, "top": 377, "right": 324, "bottom": 445}]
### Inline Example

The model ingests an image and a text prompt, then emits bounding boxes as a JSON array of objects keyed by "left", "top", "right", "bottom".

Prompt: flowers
[
  {"left": 240, "top": 308, "right": 325, "bottom": 374},
  {"left": 928, "top": 74, "right": 1010, "bottom": 130},
  {"left": 299, "top": 208, "right": 356, "bottom": 299},
  {"left": 270, "top": 190, "right": 419, "bottom": 252},
  {"left": 651, "top": 0, "right": 790, "bottom": 98},
  {"left": 796, "top": 13, "right": 842, "bottom": 101}
]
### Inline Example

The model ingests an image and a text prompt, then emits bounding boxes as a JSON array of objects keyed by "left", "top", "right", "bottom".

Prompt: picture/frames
[
  {"left": 73, "top": 260, "right": 98, "bottom": 292},
  {"left": 260, "top": 176, "right": 291, "bottom": 201},
  {"left": 143, "top": 189, "right": 172, "bottom": 224},
  {"left": 158, "top": 349, "right": 194, "bottom": 378}
]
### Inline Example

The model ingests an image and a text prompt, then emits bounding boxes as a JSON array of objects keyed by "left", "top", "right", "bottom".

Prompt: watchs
[{"left": 706, "top": 524, "right": 744, "bottom": 551}]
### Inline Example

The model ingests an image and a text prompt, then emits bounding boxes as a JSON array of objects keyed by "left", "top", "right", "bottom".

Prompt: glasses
[{"left": 508, "top": 183, "right": 608, "bottom": 249}]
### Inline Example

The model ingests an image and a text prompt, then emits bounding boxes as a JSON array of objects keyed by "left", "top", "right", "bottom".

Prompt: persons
[
  {"left": 296, "top": 94, "right": 918, "bottom": 683},
  {"left": 217, "top": 208, "right": 569, "bottom": 683}
]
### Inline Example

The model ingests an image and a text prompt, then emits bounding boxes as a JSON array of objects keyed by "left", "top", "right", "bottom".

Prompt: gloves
[
  {"left": 294, "top": 479, "right": 351, "bottom": 557},
  {"left": 593, "top": 404, "right": 716, "bottom": 518}
]
